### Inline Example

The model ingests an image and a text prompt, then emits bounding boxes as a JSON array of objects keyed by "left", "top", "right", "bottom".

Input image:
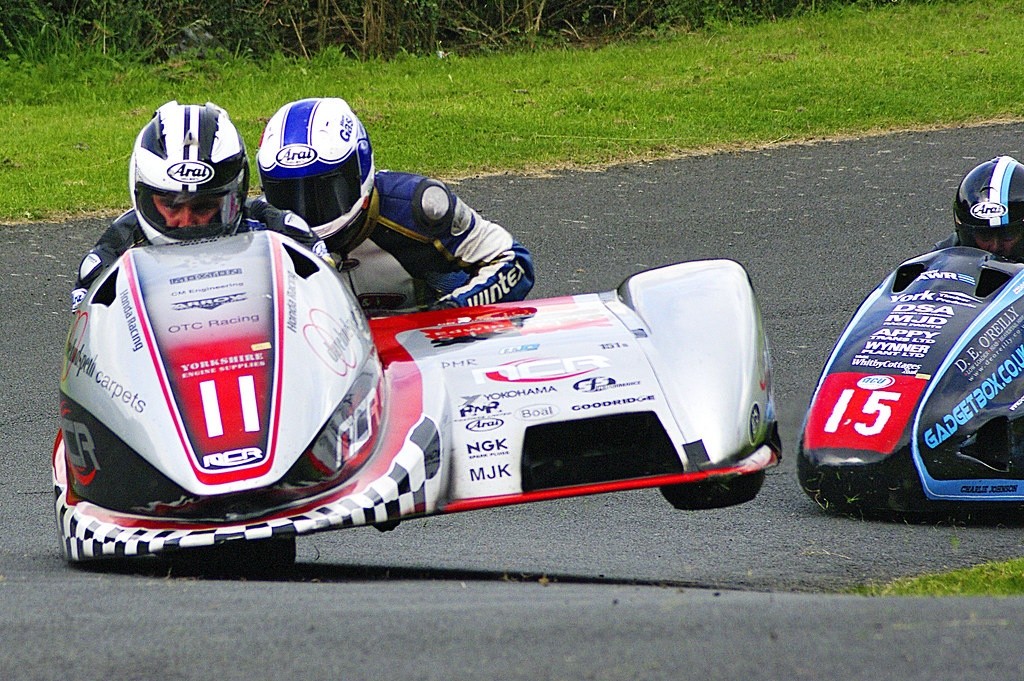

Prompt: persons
[
  {"left": 72, "top": 99, "right": 339, "bottom": 320},
  {"left": 254, "top": 95, "right": 537, "bottom": 320},
  {"left": 927, "top": 150, "right": 1024, "bottom": 274}
]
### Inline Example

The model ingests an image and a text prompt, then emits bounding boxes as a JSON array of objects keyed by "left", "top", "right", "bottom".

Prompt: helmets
[
  {"left": 129, "top": 100, "right": 250, "bottom": 245},
  {"left": 953, "top": 156, "right": 1024, "bottom": 262},
  {"left": 255, "top": 98, "right": 376, "bottom": 253}
]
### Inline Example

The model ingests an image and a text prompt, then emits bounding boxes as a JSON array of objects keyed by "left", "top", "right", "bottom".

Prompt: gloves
[{"left": 417, "top": 304, "right": 455, "bottom": 312}]
